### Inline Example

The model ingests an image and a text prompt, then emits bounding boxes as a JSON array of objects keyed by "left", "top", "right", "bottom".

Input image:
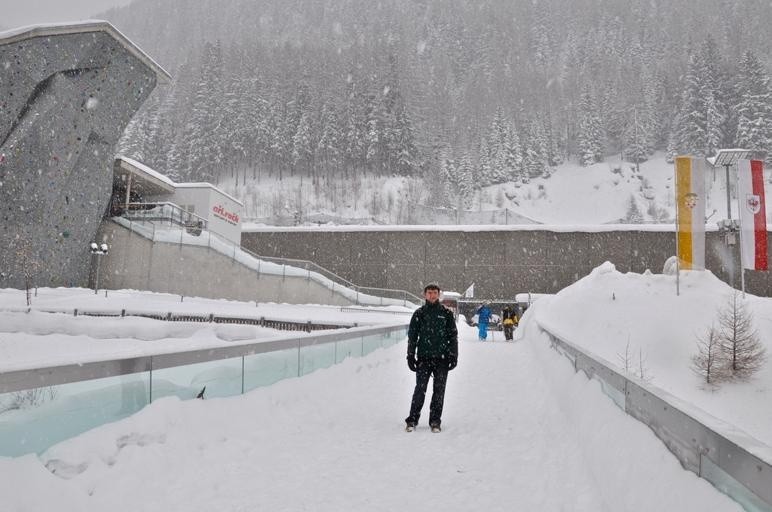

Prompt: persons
[
  {"left": 404, "top": 284, "right": 458, "bottom": 431},
  {"left": 476, "top": 297, "right": 497, "bottom": 340},
  {"left": 500, "top": 302, "right": 520, "bottom": 341}
]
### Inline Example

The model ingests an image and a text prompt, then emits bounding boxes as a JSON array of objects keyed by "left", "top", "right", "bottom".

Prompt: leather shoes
[
  {"left": 405, "top": 421, "right": 417, "bottom": 432},
  {"left": 430, "top": 421, "right": 442, "bottom": 434}
]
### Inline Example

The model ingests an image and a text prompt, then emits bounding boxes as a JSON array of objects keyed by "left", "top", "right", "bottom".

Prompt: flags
[
  {"left": 737, "top": 158, "right": 767, "bottom": 272},
  {"left": 675, "top": 157, "right": 705, "bottom": 271}
]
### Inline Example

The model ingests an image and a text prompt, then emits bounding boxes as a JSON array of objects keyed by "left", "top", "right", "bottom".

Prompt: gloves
[
  {"left": 448, "top": 356, "right": 457, "bottom": 371},
  {"left": 406, "top": 352, "right": 419, "bottom": 372}
]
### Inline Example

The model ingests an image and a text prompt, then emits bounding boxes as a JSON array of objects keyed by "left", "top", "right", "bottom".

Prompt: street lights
[{"left": 90, "top": 243, "right": 108, "bottom": 294}]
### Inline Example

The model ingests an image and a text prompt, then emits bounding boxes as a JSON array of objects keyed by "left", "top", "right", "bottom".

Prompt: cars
[{"left": 472, "top": 315, "right": 503, "bottom": 333}]
